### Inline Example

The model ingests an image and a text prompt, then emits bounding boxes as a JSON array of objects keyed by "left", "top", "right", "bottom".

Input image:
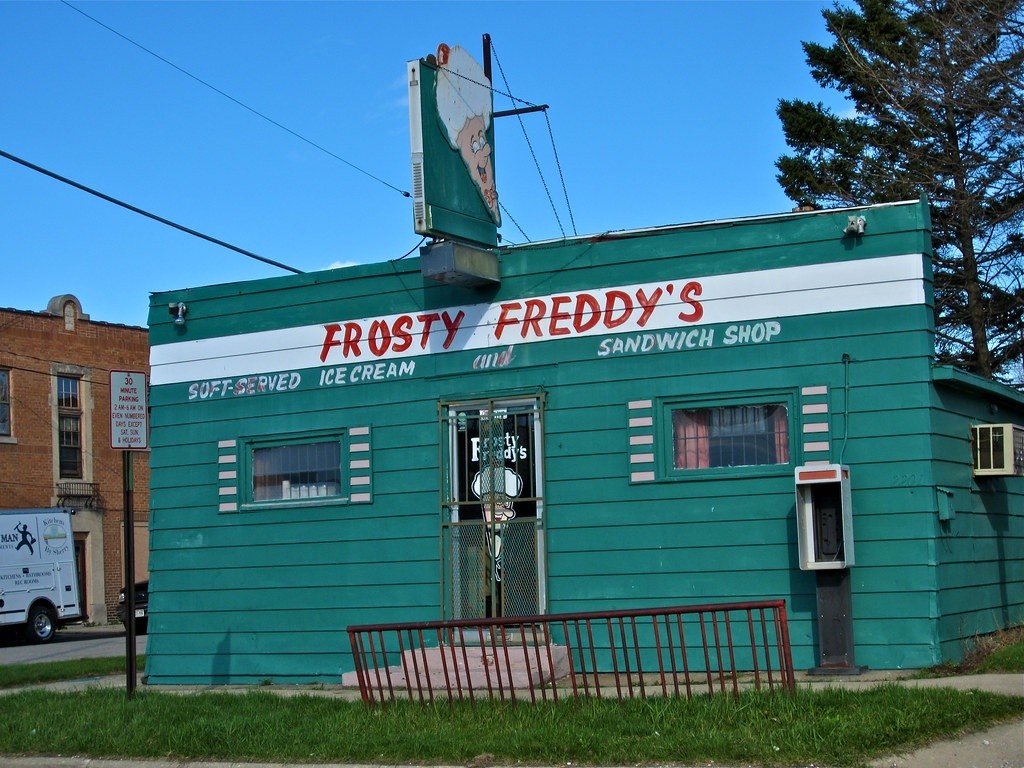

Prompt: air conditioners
[{"left": 971, "top": 423, "right": 1024, "bottom": 476}]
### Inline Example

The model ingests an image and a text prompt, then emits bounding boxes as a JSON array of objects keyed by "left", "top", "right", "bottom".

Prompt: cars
[{"left": 114, "top": 579, "right": 149, "bottom": 636}]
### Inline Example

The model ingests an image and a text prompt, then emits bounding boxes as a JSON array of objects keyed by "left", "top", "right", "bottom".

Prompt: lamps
[
  {"left": 843, "top": 218, "right": 866, "bottom": 236},
  {"left": 175, "top": 302, "right": 186, "bottom": 325}
]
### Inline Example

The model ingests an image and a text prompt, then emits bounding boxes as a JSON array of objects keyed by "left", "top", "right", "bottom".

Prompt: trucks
[{"left": 0, "top": 506, "right": 86, "bottom": 645}]
